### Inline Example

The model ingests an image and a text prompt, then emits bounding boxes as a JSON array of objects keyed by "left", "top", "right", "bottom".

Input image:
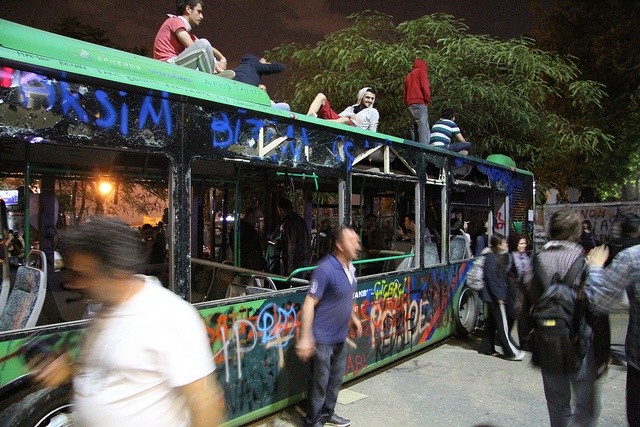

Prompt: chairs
[
  {"left": 0, "top": 249, "right": 47, "bottom": 330},
  {"left": 245, "top": 274, "right": 277, "bottom": 295},
  {"left": 449, "top": 227, "right": 468, "bottom": 261},
  {"left": 422, "top": 234, "right": 441, "bottom": 267},
  {"left": 191, "top": 257, "right": 215, "bottom": 302},
  {"left": 206, "top": 261, "right": 234, "bottom": 300},
  {"left": 388, "top": 240, "right": 414, "bottom": 273},
  {"left": 0, "top": 259, "right": 12, "bottom": 319}
]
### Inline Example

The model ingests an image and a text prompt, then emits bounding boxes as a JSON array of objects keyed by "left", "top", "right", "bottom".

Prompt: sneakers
[
  {"left": 480, "top": 349, "right": 501, "bottom": 357},
  {"left": 216, "top": 70, "right": 236, "bottom": 78},
  {"left": 507, "top": 351, "right": 525, "bottom": 361},
  {"left": 325, "top": 415, "right": 351, "bottom": 427}
]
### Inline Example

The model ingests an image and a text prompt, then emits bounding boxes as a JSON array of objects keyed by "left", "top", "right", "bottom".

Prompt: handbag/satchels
[{"left": 509, "top": 275, "right": 525, "bottom": 320}]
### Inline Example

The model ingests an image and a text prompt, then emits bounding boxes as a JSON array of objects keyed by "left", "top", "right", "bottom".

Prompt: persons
[
  {"left": 320, "top": 218, "right": 331, "bottom": 235},
  {"left": 584, "top": 215, "right": 640, "bottom": 427},
  {"left": 232, "top": 55, "right": 287, "bottom": 87},
  {"left": 295, "top": 227, "right": 363, "bottom": 427},
  {"left": 154, "top": 0, "right": 236, "bottom": 79},
  {"left": 563, "top": 183, "right": 581, "bottom": 203},
  {"left": 267, "top": 198, "right": 312, "bottom": 280},
  {"left": 545, "top": 184, "right": 561, "bottom": 204},
  {"left": 508, "top": 233, "right": 535, "bottom": 351},
  {"left": 395, "top": 213, "right": 431, "bottom": 242},
  {"left": 28, "top": 216, "right": 226, "bottom": 426},
  {"left": 481, "top": 233, "right": 525, "bottom": 361},
  {"left": 529, "top": 208, "right": 610, "bottom": 427},
  {"left": 139, "top": 221, "right": 168, "bottom": 264},
  {"left": 258, "top": 84, "right": 290, "bottom": 111},
  {"left": 582, "top": 220, "right": 597, "bottom": 247},
  {"left": 306, "top": 87, "right": 380, "bottom": 132},
  {"left": 431, "top": 109, "right": 472, "bottom": 155},
  {"left": 455, "top": 211, "right": 474, "bottom": 257},
  {"left": 404, "top": 58, "right": 431, "bottom": 145},
  {"left": 5, "top": 230, "right": 25, "bottom": 260},
  {"left": 229, "top": 205, "right": 269, "bottom": 272},
  {"left": 364, "top": 213, "right": 390, "bottom": 249},
  {"left": 32, "top": 236, "right": 65, "bottom": 273}
]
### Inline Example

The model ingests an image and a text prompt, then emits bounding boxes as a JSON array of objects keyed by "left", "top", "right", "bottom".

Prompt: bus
[{"left": 0, "top": 21, "right": 536, "bottom": 427}]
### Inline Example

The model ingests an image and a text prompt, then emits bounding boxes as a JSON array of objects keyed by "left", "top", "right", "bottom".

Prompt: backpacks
[
  {"left": 466, "top": 246, "right": 492, "bottom": 291},
  {"left": 529, "top": 252, "right": 588, "bottom": 378}
]
